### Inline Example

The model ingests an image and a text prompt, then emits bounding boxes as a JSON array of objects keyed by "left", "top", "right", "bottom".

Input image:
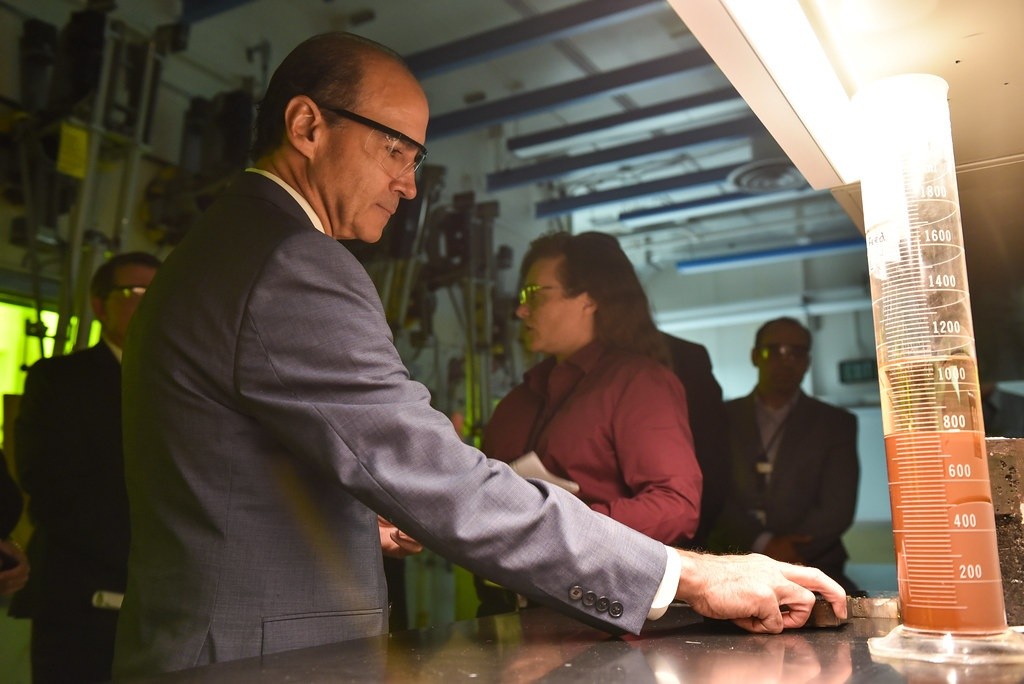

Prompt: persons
[
  {"left": 13, "top": 251, "right": 162, "bottom": 684},
  {"left": 717, "top": 316, "right": 868, "bottom": 599},
  {"left": 0, "top": 447, "right": 23, "bottom": 545},
  {"left": 112, "top": 33, "right": 850, "bottom": 684},
  {"left": 573, "top": 231, "right": 735, "bottom": 551},
  {"left": 475, "top": 229, "right": 703, "bottom": 605}
]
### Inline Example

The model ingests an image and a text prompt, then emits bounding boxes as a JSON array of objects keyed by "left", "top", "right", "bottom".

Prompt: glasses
[
  {"left": 755, "top": 341, "right": 811, "bottom": 364},
  {"left": 515, "top": 282, "right": 568, "bottom": 310},
  {"left": 100, "top": 282, "right": 152, "bottom": 303},
  {"left": 312, "top": 102, "right": 427, "bottom": 181}
]
